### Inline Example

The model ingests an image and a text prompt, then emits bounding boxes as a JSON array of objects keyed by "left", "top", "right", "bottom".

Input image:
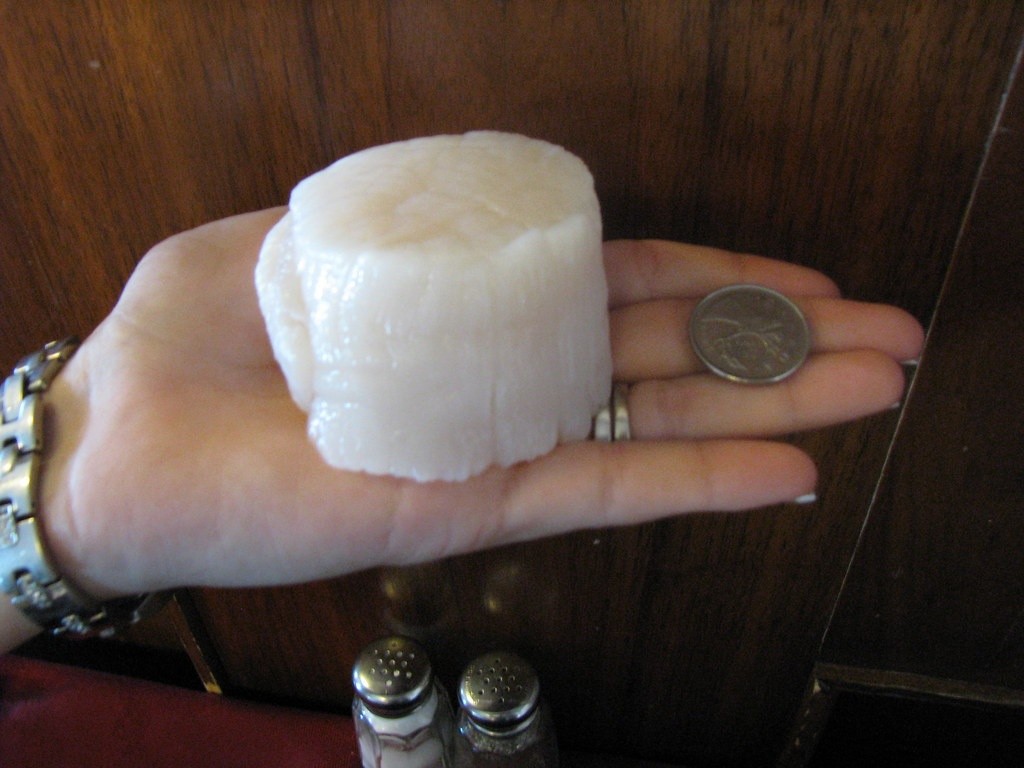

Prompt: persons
[{"left": 0, "top": 204, "right": 926, "bottom": 655}]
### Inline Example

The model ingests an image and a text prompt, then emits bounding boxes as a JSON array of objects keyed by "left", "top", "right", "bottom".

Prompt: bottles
[{"left": 350, "top": 636, "right": 547, "bottom": 768}]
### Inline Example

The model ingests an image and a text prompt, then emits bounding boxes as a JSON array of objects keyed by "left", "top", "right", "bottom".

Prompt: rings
[{"left": 593, "top": 381, "right": 631, "bottom": 440}]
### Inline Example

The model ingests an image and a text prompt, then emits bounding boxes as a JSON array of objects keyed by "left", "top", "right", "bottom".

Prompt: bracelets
[{"left": 0, "top": 338, "right": 166, "bottom": 637}]
